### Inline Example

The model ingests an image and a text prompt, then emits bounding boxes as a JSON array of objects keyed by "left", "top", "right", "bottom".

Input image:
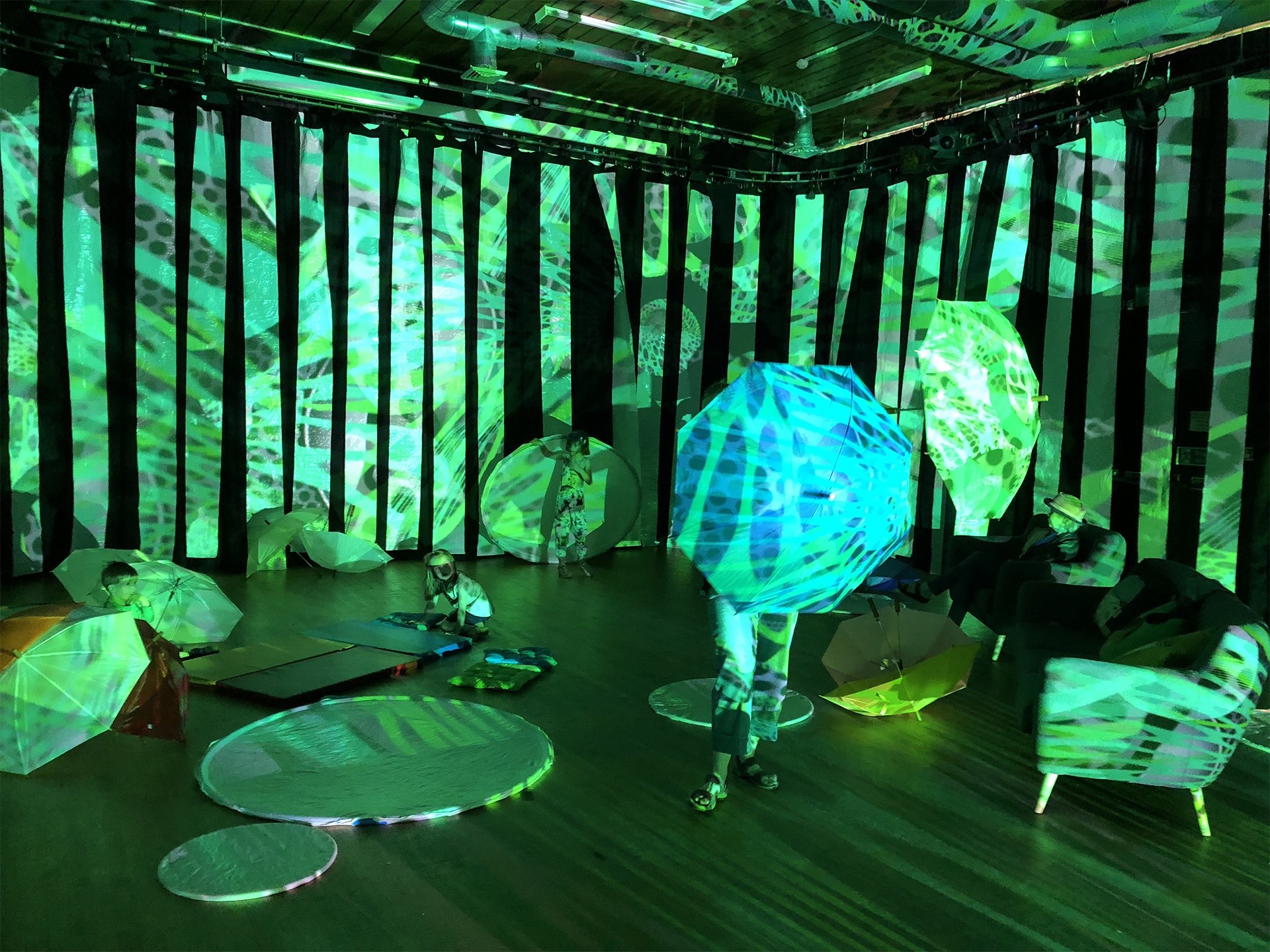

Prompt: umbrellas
[
  {"left": 246, "top": 508, "right": 327, "bottom": 579},
  {"left": 666, "top": 360, "right": 914, "bottom": 616},
  {"left": 128, "top": 560, "right": 244, "bottom": 645},
  {"left": 0, "top": 606, "right": 152, "bottom": 775},
  {"left": 886, "top": 298, "right": 1049, "bottom": 521},
  {"left": 0, "top": 602, "right": 85, "bottom": 671},
  {"left": 819, "top": 594, "right": 984, "bottom": 721},
  {"left": 298, "top": 505, "right": 395, "bottom": 578},
  {"left": 246, "top": 505, "right": 284, "bottom": 542},
  {"left": 50, "top": 549, "right": 151, "bottom": 608},
  {"left": 110, "top": 618, "right": 190, "bottom": 746}
]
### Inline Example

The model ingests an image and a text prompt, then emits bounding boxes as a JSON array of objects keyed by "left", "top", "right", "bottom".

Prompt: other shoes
[
  {"left": 189, "top": 645, "right": 220, "bottom": 657},
  {"left": 575, "top": 558, "right": 594, "bottom": 578},
  {"left": 465, "top": 627, "right": 489, "bottom": 638},
  {"left": 556, "top": 563, "right": 574, "bottom": 579}
]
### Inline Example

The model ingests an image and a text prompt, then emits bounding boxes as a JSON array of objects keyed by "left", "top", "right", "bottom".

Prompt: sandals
[
  {"left": 736, "top": 755, "right": 779, "bottom": 791},
  {"left": 689, "top": 771, "right": 728, "bottom": 812}
]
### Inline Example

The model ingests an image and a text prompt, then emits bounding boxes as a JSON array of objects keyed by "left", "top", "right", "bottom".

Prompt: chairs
[
  {"left": 1033, "top": 556, "right": 1270, "bottom": 838},
  {"left": 947, "top": 513, "right": 1127, "bottom": 662}
]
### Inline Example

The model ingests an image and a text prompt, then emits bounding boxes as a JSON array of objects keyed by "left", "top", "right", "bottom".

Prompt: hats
[{"left": 1043, "top": 492, "right": 1089, "bottom": 526}]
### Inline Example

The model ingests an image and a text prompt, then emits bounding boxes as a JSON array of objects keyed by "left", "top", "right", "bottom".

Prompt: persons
[
  {"left": 688, "top": 382, "right": 798, "bottom": 812},
  {"left": 530, "top": 431, "right": 594, "bottom": 578},
  {"left": 100, "top": 560, "right": 153, "bottom": 625},
  {"left": 424, "top": 548, "right": 494, "bottom": 636},
  {"left": 898, "top": 491, "right": 1088, "bottom": 627}
]
